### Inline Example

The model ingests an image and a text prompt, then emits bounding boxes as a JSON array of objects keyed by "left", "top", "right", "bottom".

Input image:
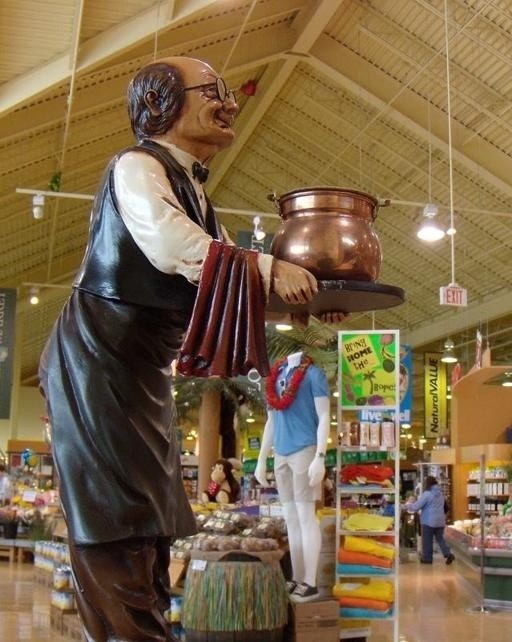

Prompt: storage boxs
[{"left": 292, "top": 595, "right": 340, "bottom": 642}]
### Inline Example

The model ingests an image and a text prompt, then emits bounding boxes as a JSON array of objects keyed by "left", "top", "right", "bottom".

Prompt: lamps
[
  {"left": 443, "top": 339, "right": 455, "bottom": 349},
  {"left": 21, "top": 281, "right": 73, "bottom": 306},
  {"left": 440, "top": 349, "right": 458, "bottom": 363},
  {"left": 14, "top": 180, "right": 280, "bottom": 242},
  {"left": 416, "top": 91, "right": 444, "bottom": 242}
]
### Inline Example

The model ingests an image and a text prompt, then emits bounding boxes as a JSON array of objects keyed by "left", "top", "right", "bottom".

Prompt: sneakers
[
  {"left": 285, "top": 579, "right": 297, "bottom": 595},
  {"left": 420, "top": 558, "right": 434, "bottom": 564},
  {"left": 291, "top": 584, "right": 319, "bottom": 604},
  {"left": 445, "top": 555, "right": 455, "bottom": 564}
]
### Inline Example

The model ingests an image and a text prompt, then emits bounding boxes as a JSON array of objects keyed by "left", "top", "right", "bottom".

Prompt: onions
[{"left": 369, "top": 394, "right": 384, "bottom": 405}]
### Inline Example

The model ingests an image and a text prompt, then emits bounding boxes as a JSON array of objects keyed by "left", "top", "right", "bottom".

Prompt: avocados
[
  {"left": 356, "top": 397, "right": 367, "bottom": 405},
  {"left": 383, "top": 360, "right": 395, "bottom": 373}
]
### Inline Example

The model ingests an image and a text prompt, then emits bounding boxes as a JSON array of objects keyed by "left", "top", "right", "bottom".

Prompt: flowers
[
  {"left": 265, "top": 354, "right": 314, "bottom": 409},
  {"left": 0, "top": 485, "right": 44, "bottom": 539}
]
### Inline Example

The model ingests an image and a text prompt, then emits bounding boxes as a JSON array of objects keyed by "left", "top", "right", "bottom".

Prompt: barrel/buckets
[{"left": 180, "top": 554, "right": 292, "bottom": 641}]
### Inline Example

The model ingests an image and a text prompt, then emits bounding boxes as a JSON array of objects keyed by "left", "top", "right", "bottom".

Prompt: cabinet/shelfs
[
  {"left": 442, "top": 517, "right": 512, "bottom": 613},
  {"left": 465, "top": 479, "right": 511, "bottom": 515}
]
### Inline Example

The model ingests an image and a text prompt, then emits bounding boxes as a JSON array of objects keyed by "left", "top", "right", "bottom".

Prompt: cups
[{"left": 358, "top": 419, "right": 395, "bottom": 448}]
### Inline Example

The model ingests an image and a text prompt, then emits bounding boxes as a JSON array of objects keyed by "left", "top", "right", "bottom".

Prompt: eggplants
[{"left": 344, "top": 383, "right": 354, "bottom": 402}]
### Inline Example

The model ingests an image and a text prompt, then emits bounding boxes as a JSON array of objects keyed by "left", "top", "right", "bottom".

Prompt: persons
[
  {"left": 400, "top": 476, "right": 455, "bottom": 564},
  {"left": 404, "top": 495, "right": 422, "bottom": 514},
  {"left": 254, "top": 348, "right": 334, "bottom": 603},
  {"left": 37, "top": 59, "right": 350, "bottom": 641},
  {"left": 0, "top": 464, "right": 16, "bottom": 539}
]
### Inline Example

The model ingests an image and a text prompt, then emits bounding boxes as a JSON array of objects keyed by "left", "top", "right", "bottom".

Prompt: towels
[{"left": 331, "top": 463, "right": 396, "bottom": 619}]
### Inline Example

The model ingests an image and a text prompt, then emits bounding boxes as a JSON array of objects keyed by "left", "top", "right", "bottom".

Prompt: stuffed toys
[{"left": 201, "top": 458, "right": 244, "bottom": 503}]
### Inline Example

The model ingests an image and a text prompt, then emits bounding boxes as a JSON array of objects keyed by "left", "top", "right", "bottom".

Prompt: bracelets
[{"left": 315, "top": 451, "right": 326, "bottom": 458}]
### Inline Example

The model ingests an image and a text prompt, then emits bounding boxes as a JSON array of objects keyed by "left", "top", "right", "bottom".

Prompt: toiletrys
[{"left": 342, "top": 416, "right": 396, "bottom": 449}]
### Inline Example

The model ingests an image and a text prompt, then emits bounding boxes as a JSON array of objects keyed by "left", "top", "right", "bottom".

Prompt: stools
[
  {"left": 0, "top": 539, "right": 14, "bottom": 564},
  {"left": 14, "top": 539, "right": 35, "bottom": 562}
]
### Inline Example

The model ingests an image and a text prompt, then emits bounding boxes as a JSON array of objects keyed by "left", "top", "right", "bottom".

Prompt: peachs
[{"left": 381, "top": 334, "right": 393, "bottom": 345}]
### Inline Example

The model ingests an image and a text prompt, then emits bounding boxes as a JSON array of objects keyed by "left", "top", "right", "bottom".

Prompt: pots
[{"left": 265, "top": 187, "right": 394, "bottom": 286}]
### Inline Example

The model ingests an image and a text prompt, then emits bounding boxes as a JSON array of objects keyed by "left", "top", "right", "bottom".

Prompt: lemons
[{"left": 384, "top": 397, "right": 395, "bottom": 405}]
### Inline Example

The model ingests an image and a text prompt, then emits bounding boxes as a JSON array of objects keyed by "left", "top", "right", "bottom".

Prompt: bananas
[{"left": 382, "top": 345, "right": 395, "bottom": 360}]
